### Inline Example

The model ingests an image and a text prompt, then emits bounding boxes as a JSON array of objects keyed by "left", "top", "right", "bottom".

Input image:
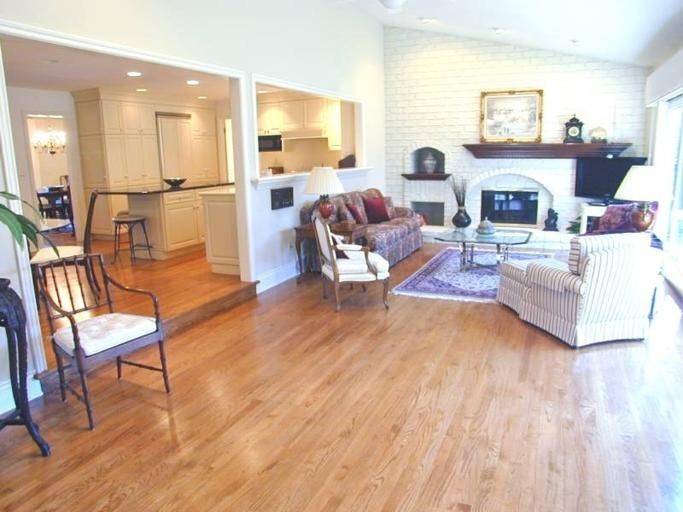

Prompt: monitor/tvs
[{"left": 574, "top": 157, "right": 649, "bottom": 206}]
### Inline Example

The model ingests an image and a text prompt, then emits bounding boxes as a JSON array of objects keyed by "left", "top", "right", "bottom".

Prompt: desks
[
  {"left": 25, "top": 217, "right": 70, "bottom": 301},
  {"left": 0, "top": 279, "right": 50, "bottom": 459},
  {"left": 294, "top": 222, "right": 369, "bottom": 283},
  {"left": 579, "top": 202, "right": 606, "bottom": 234}
]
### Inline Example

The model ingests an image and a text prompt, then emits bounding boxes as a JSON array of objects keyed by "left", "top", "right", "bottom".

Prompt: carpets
[{"left": 388, "top": 246, "right": 554, "bottom": 306}]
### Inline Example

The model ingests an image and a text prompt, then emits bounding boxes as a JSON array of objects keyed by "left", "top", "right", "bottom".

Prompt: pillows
[
  {"left": 338, "top": 201, "right": 353, "bottom": 221},
  {"left": 363, "top": 196, "right": 389, "bottom": 224},
  {"left": 348, "top": 204, "right": 367, "bottom": 225}
]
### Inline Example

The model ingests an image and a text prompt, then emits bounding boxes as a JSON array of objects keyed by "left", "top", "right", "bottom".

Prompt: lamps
[
  {"left": 31, "top": 118, "right": 66, "bottom": 158},
  {"left": 303, "top": 164, "right": 345, "bottom": 219},
  {"left": 610, "top": 163, "right": 677, "bottom": 232}
]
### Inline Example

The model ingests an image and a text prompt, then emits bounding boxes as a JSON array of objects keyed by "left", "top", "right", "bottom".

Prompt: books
[{"left": 44, "top": 185, "right": 64, "bottom": 192}]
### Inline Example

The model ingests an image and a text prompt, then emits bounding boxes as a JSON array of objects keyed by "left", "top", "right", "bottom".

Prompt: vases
[
  {"left": 422, "top": 152, "right": 437, "bottom": 173},
  {"left": 451, "top": 207, "right": 471, "bottom": 228}
]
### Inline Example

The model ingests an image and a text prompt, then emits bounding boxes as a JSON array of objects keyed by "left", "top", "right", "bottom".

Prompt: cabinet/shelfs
[
  {"left": 190, "top": 106, "right": 218, "bottom": 181},
  {"left": 78, "top": 136, "right": 125, "bottom": 185},
  {"left": 124, "top": 99, "right": 156, "bottom": 136},
  {"left": 77, "top": 100, "right": 124, "bottom": 136},
  {"left": 256, "top": 102, "right": 281, "bottom": 136},
  {"left": 281, "top": 97, "right": 326, "bottom": 140},
  {"left": 84, "top": 186, "right": 127, "bottom": 240},
  {"left": 127, "top": 186, "right": 223, "bottom": 261},
  {"left": 125, "top": 135, "right": 160, "bottom": 184},
  {"left": 323, "top": 99, "right": 356, "bottom": 150},
  {"left": 198, "top": 186, "right": 239, "bottom": 276}
]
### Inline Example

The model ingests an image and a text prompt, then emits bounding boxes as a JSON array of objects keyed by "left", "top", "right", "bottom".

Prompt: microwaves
[{"left": 256, "top": 134, "right": 282, "bottom": 153}]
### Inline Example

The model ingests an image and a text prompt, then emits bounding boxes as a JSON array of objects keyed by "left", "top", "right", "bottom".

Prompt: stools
[{"left": 112, "top": 214, "right": 154, "bottom": 266}]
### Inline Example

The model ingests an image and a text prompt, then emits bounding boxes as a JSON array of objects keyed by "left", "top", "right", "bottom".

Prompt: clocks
[{"left": 562, "top": 113, "right": 585, "bottom": 144}]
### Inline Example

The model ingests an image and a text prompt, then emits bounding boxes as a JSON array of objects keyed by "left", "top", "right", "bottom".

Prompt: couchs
[
  {"left": 526, "top": 233, "right": 662, "bottom": 349},
  {"left": 298, "top": 187, "right": 423, "bottom": 270},
  {"left": 586, "top": 201, "right": 660, "bottom": 234},
  {"left": 499, "top": 255, "right": 568, "bottom": 311}
]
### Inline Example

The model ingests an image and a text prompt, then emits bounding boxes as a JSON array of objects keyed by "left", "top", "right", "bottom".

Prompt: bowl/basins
[
  {"left": 161, "top": 178, "right": 185, "bottom": 187},
  {"left": 43, "top": 185, "right": 64, "bottom": 191}
]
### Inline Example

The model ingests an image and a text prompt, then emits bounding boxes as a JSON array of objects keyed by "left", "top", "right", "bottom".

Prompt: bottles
[{"left": 475, "top": 215, "right": 496, "bottom": 235}]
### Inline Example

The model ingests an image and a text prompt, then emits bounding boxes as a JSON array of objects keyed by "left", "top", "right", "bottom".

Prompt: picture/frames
[{"left": 479, "top": 90, "right": 542, "bottom": 144}]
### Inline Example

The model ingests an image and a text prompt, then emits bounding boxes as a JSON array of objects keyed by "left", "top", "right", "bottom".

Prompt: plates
[{"left": 588, "top": 127, "right": 607, "bottom": 140}]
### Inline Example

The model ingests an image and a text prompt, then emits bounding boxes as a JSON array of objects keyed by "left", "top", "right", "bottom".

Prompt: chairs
[
  {"left": 37, "top": 190, "right": 64, "bottom": 218},
  {"left": 34, "top": 251, "right": 170, "bottom": 432},
  {"left": 308, "top": 209, "right": 392, "bottom": 311},
  {"left": 30, "top": 188, "right": 102, "bottom": 310},
  {"left": 59, "top": 175, "right": 69, "bottom": 190}
]
